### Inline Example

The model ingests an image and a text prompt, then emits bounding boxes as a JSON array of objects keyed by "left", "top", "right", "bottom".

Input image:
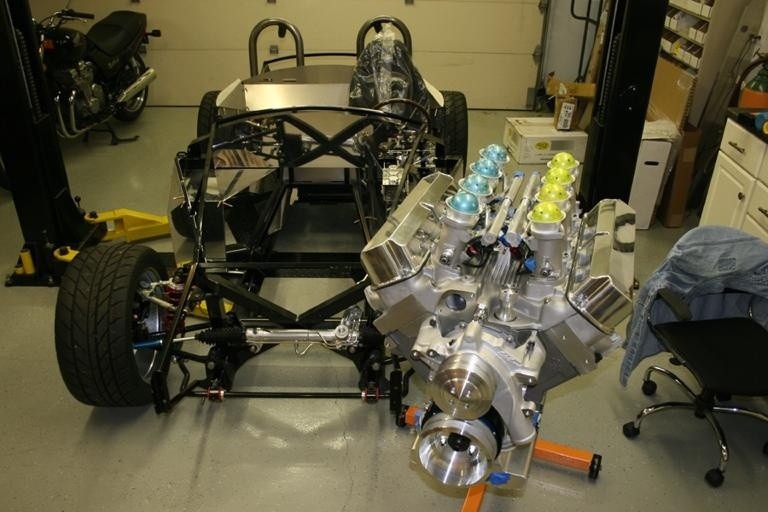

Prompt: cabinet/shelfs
[
  {"left": 656, "top": 1, "right": 750, "bottom": 78},
  {"left": 697, "top": 119, "right": 768, "bottom": 242}
]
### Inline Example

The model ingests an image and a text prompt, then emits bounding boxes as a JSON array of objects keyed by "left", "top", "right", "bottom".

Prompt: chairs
[{"left": 622, "top": 224, "right": 768, "bottom": 489}]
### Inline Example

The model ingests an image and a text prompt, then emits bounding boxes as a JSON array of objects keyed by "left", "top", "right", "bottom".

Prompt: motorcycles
[{"left": 1, "top": 9, "right": 160, "bottom": 193}]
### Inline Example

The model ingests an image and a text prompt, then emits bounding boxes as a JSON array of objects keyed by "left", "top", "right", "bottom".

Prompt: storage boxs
[{"left": 504, "top": 70, "right": 598, "bottom": 167}]
